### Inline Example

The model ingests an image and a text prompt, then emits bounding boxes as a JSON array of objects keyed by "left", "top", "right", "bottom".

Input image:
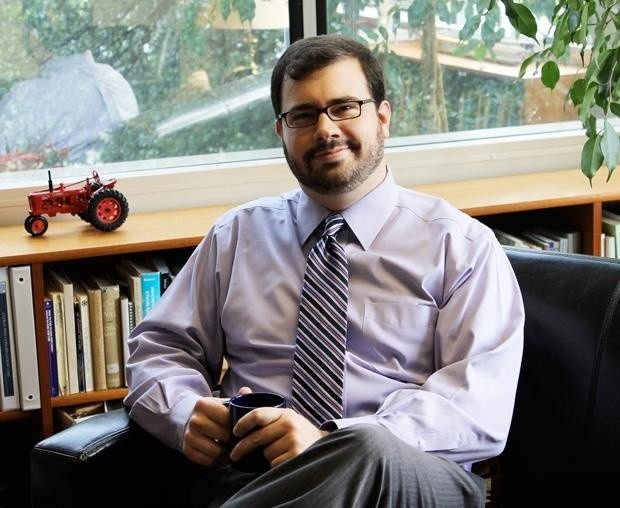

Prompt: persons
[
  {"left": 124, "top": 33, "right": 528, "bottom": 506},
  {"left": 1, "top": 2, "right": 151, "bottom": 159}
]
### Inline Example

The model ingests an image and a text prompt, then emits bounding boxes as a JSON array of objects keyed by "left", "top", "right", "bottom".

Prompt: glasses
[{"left": 277, "top": 97, "right": 378, "bottom": 129}]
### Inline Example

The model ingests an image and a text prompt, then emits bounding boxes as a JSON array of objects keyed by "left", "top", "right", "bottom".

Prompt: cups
[{"left": 219, "top": 391, "right": 286, "bottom": 469}]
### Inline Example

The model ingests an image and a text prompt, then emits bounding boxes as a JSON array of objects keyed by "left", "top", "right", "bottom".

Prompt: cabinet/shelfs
[
  {"left": 0, "top": 164, "right": 620, "bottom": 438},
  {"left": 369, "top": 40, "right": 592, "bottom": 137}
]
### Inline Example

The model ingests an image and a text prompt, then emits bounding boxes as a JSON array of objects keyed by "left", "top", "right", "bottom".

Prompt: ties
[{"left": 290, "top": 211, "right": 352, "bottom": 429}]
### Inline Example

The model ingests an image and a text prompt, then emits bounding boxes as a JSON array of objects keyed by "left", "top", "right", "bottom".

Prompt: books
[
  {"left": 600, "top": 208, "right": 620, "bottom": 260},
  {"left": 43, "top": 249, "right": 182, "bottom": 399},
  {"left": 477, "top": 214, "right": 582, "bottom": 256},
  {"left": 386, "top": 56, "right": 526, "bottom": 129}
]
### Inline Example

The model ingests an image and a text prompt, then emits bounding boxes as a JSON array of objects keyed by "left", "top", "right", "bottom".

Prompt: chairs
[
  {"left": 31, "top": 246, "right": 620, "bottom": 508},
  {"left": 105, "top": 72, "right": 278, "bottom": 160}
]
